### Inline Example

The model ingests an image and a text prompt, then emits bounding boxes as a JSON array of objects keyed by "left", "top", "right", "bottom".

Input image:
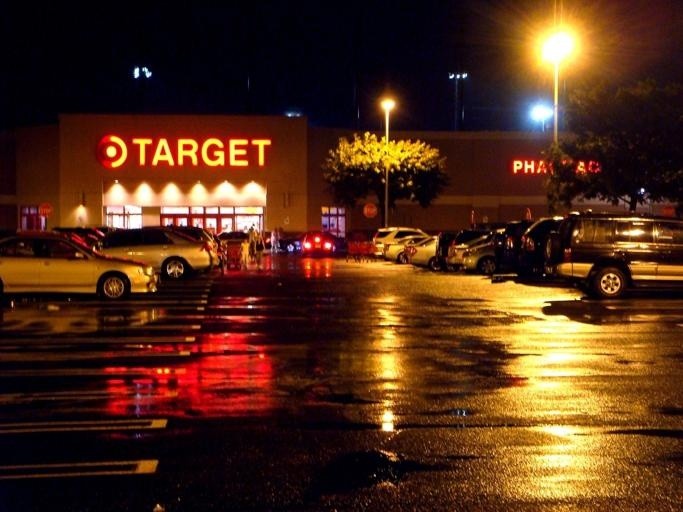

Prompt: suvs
[{"left": 550, "top": 210, "right": 682, "bottom": 298}]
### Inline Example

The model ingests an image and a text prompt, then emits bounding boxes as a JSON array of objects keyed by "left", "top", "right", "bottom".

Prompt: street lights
[
  {"left": 132, "top": 66, "right": 153, "bottom": 94},
  {"left": 542, "top": 28, "right": 574, "bottom": 146},
  {"left": 382, "top": 99, "right": 395, "bottom": 227},
  {"left": 448, "top": 72, "right": 468, "bottom": 131}
]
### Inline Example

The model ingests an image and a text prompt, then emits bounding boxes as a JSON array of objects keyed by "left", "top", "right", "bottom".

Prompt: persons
[
  {"left": 247, "top": 223, "right": 264, "bottom": 270},
  {"left": 269, "top": 226, "right": 280, "bottom": 256},
  {"left": 237, "top": 238, "right": 251, "bottom": 271}
]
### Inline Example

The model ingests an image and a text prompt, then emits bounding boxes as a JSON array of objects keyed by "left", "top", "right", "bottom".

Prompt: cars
[
  {"left": 0, "top": 231, "right": 163, "bottom": 302},
  {"left": 49, "top": 223, "right": 340, "bottom": 265},
  {"left": 94, "top": 227, "right": 221, "bottom": 282},
  {"left": 347, "top": 215, "right": 565, "bottom": 280}
]
